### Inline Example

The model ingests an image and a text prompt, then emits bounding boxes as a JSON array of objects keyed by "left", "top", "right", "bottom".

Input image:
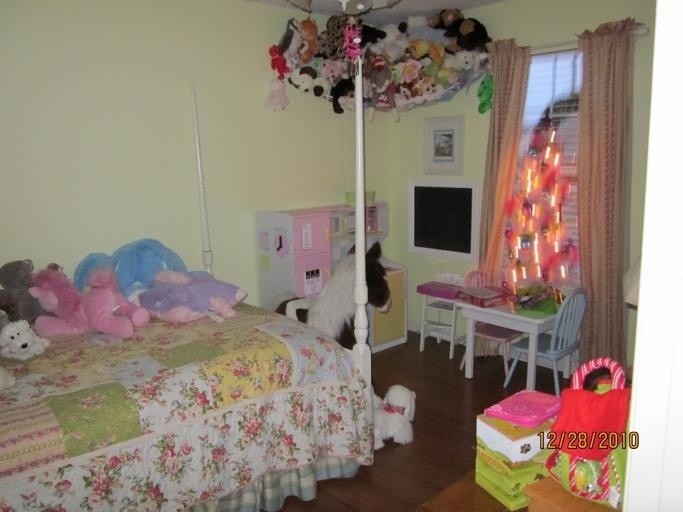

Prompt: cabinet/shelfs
[
  {"left": 255, "top": 204, "right": 408, "bottom": 353},
  {"left": 412, "top": 281, "right": 468, "bottom": 363}
]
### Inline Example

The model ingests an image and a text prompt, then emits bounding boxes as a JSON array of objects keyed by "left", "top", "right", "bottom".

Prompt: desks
[{"left": 456, "top": 297, "right": 576, "bottom": 392}]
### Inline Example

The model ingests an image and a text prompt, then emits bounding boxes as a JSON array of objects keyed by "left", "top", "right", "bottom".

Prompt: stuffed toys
[
  {"left": 274, "top": 241, "right": 391, "bottom": 348},
  {"left": 267, "top": 0, "right": 495, "bottom": 113},
  {"left": 372, "top": 385, "right": 417, "bottom": 450},
  {"left": 0, "top": 239, "right": 250, "bottom": 361}
]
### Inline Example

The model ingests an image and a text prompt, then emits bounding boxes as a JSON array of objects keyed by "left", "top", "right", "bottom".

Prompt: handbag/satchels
[{"left": 542, "top": 356, "right": 631, "bottom": 510}]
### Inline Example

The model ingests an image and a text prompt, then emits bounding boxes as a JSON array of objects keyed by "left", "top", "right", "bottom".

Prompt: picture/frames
[{"left": 422, "top": 115, "right": 465, "bottom": 177}]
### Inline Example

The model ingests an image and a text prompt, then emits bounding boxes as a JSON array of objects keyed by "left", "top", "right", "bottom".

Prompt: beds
[{"left": 0, "top": 44, "right": 374, "bottom": 512}]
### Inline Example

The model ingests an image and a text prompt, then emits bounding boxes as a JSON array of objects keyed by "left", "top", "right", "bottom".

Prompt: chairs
[
  {"left": 456, "top": 267, "right": 528, "bottom": 381},
  {"left": 503, "top": 286, "right": 590, "bottom": 399}
]
